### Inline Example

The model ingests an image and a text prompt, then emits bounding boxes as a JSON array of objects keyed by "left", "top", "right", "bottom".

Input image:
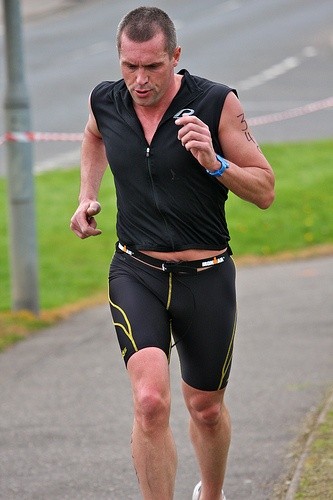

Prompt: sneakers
[{"left": 191, "top": 480, "right": 227, "bottom": 500}]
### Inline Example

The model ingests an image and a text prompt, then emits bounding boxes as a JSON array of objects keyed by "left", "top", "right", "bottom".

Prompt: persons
[{"left": 69, "top": 7, "right": 275, "bottom": 500}]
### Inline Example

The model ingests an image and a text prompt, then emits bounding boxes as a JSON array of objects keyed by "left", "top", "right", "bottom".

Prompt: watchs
[{"left": 206, "top": 155, "right": 229, "bottom": 178}]
